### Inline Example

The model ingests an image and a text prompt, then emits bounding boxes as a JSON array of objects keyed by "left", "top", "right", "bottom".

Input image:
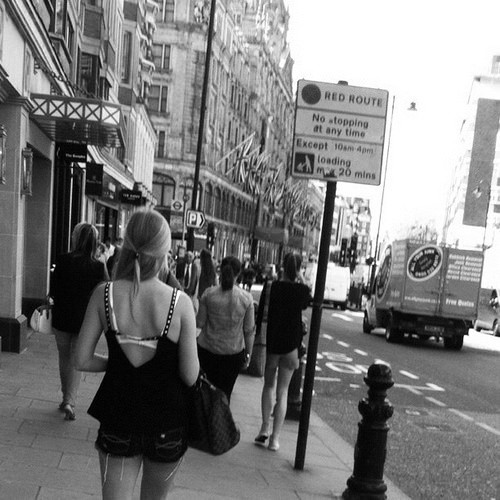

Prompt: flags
[{"left": 231, "top": 134, "right": 322, "bottom": 230}]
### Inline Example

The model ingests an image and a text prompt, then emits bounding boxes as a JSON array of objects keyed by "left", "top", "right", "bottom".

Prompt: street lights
[
  {"left": 369, "top": 96, "right": 419, "bottom": 293},
  {"left": 334, "top": 206, "right": 355, "bottom": 245},
  {"left": 249, "top": 167, "right": 279, "bottom": 270}
]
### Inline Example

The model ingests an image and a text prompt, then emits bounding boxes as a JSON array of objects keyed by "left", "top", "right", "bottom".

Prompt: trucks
[{"left": 361, "top": 239, "right": 485, "bottom": 350}]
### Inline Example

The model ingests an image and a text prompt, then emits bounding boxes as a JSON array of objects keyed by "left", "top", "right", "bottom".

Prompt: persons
[
  {"left": 195, "top": 255, "right": 256, "bottom": 405},
  {"left": 176, "top": 247, "right": 277, "bottom": 309},
  {"left": 349, "top": 275, "right": 367, "bottom": 311},
  {"left": 96, "top": 234, "right": 125, "bottom": 284},
  {"left": 254, "top": 251, "right": 313, "bottom": 452},
  {"left": 47, "top": 223, "right": 111, "bottom": 422},
  {"left": 73, "top": 211, "right": 200, "bottom": 500}
]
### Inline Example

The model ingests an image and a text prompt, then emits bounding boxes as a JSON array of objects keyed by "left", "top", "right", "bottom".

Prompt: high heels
[
  {"left": 58, "top": 400, "right": 66, "bottom": 409},
  {"left": 63, "top": 402, "right": 77, "bottom": 420}
]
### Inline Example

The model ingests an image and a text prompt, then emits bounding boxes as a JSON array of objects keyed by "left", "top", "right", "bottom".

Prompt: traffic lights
[
  {"left": 339, "top": 238, "right": 348, "bottom": 266},
  {"left": 206, "top": 223, "right": 214, "bottom": 250},
  {"left": 347, "top": 237, "right": 357, "bottom": 268}
]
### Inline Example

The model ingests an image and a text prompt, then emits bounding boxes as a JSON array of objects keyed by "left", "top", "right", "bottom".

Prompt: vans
[
  {"left": 474, "top": 285, "right": 500, "bottom": 338},
  {"left": 304, "top": 264, "right": 351, "bottom": 310}
]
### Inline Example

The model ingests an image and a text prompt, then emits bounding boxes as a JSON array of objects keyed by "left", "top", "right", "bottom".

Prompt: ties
[{"left": 185, "top": 264, "right": 190, "bottom": 287}]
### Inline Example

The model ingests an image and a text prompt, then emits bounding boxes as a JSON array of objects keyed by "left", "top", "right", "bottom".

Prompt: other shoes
[
  {"left": 255, "top": 433, "right": 268, "bottom": 443},
  {"left": 269, "top": 441, "right": 279, "bottom": 451}
]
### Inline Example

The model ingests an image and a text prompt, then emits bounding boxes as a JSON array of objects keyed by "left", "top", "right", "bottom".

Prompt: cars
[{"left": 256, "top": 264, "right": 277, "bottom": 284}]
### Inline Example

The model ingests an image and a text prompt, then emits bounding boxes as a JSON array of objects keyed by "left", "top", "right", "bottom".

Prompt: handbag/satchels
[
  {"left": 30, "top": 303, "right": 53, "bottom": 335},
  {"left": 247, "top": 335, "right": 267, "bottom": 377},
  {"left": 188, "top": 369, "right": 240, "bottom": 456}
]
